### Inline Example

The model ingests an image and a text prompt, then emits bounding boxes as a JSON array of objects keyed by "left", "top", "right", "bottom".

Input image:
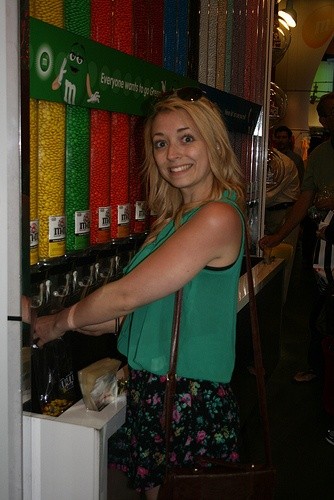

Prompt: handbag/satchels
[{"left": 157, "top": 462, "right": 278, "bottom": 499}]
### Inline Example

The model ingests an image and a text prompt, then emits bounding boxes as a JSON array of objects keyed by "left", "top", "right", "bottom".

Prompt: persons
[
  {"left": 258, "top": 93, "right": 334, "bottom": 445},
  {"left": 33, "top": 86, "right": 276, "bottom": 500}
]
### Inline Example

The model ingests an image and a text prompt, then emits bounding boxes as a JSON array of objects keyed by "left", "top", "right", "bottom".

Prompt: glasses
[{"left": 154, "top": 87, "right": 214, "bottom": 103}]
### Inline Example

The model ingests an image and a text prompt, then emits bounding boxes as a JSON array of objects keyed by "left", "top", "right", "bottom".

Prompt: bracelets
[{"left": 115, "top": 318, "right": 119, "bottom": 336}]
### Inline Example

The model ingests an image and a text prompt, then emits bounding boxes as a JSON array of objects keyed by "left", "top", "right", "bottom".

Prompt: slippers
[{"left": 293, "top": 370, "right": 320, "bottom": 383}]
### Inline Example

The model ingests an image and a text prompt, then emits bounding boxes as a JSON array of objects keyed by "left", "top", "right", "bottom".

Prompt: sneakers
[{"left": 324, "top": 429, "right": 334, "bottom": 446}]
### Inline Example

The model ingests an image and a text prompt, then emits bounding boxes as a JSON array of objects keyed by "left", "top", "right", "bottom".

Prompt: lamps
[{"left": 276, "top": 8, "right": 297, "bottom": 36}]
